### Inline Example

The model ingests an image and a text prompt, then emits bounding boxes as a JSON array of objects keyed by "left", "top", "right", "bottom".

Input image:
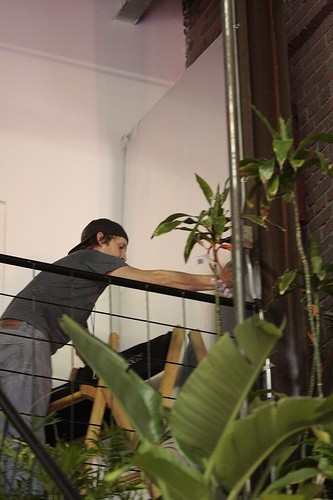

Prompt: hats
[{"left": 67, "top": 218, "right": 129, "bottom": 256}]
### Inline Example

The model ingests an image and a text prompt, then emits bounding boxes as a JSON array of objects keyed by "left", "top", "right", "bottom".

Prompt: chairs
[{"left": 42, "top": 324, "right": 185, "bottom": 500}]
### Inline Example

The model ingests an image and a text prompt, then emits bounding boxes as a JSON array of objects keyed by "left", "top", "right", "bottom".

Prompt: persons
[{"left": 1, "top": 219, "right": 247, "bottom": 500}]
